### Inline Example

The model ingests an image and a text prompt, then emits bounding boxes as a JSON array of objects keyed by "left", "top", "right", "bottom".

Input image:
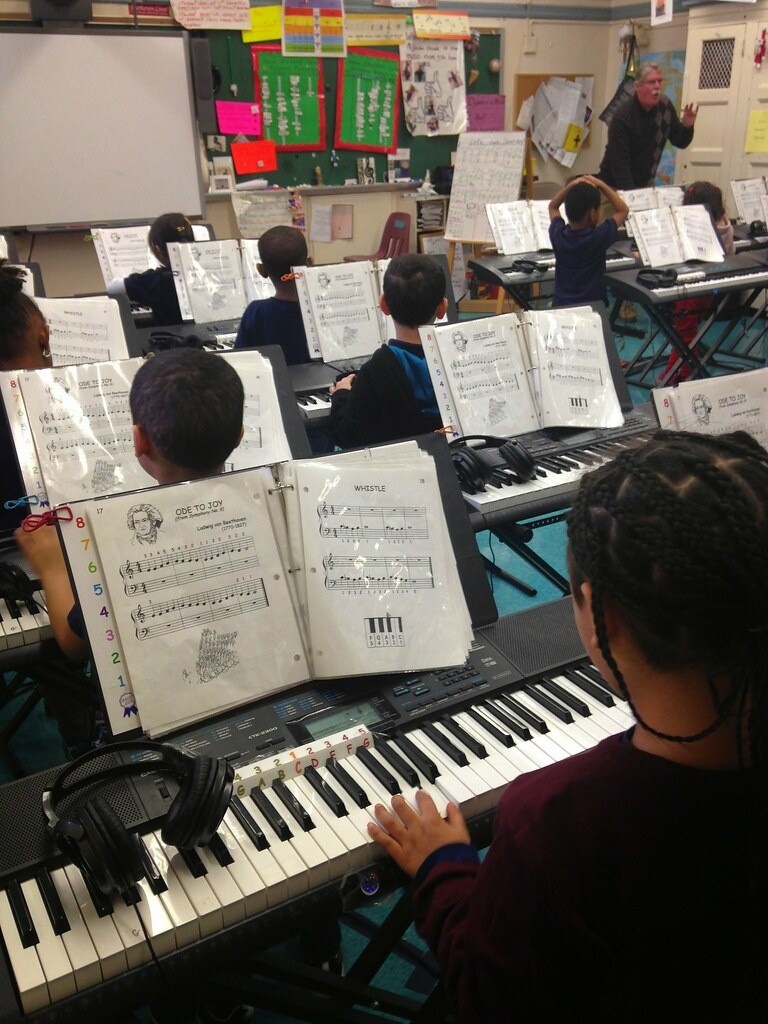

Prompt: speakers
[{"left": 190, "top": 38, "right": 218, "bottom": 134}]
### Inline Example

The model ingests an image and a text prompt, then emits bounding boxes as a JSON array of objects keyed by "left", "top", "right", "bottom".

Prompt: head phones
[
  {"left": 511, "top": 260, "right": 549, "bottom": 273},
  {"left": 636, "top": 267, "right": 677, "bottom": 289},
  {"left": 0, "top": 536, "right": 38, "bottom": 601},
  {"left": 749, "top": 220, "right": 768, "bottom": 236},
  {"left": 42, "top": 741, "right": 237, "bottom": 895},
  {"left": 149, "top": 331, "right": 204, "bottom": 352},
  {"left": 449, "top": 435, "right": 538, "bottom": 491}
]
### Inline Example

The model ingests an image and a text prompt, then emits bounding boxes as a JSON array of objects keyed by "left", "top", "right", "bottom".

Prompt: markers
[
  {"left": 44, "top": 225, "right": 66, "bottom": 229},
  {"left": 111, "top": 221, "right": 148, "bottom": 226},
  {"left": 70, "top": 224, "right": 86, "bottom": 228}
]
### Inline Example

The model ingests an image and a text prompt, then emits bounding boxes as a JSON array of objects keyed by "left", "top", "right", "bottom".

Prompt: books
[
  {"left": 0, "top": 350, "right": 293, "bottom": 515},
  {"left": 55, "top": 440, "right": 475, "bottom": 740},
  {"left": 165, "top": 239, "right": 276, "bottom": 324},
  {"left": 417, "top": 200, "right": 444, "bottom": 229},
  {"left": 91, "top": 226, "right": 210, "bottom": 293},
  {"left": 418, "top": 305, "right": 625, "bottom": 444},
  {"left": 485, "top": 176, "right": 768, "bottom": 268},
  {"left": 293, "top": 258, "right": 448, "bottom": 363},
  {"left": 652, "top": 367, "right": 768, "bottom": 456},
  {"left": 0, "top": 235, "right": 130, "bottom": 367}
]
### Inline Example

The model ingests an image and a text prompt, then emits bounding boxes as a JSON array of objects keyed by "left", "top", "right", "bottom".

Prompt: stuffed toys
[{"left": 754, "top": 30, "right": 766, "bottom": 68}]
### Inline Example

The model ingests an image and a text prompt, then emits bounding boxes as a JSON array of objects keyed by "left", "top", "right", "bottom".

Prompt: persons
[
  {"left": 14, "top": 348, "right": 244, "bottom": 763},
  {"left": 124, "top": 212, "right": 195, "bottom": 326},
  {"left": 207, "top": 137, "right": 222, "bottom": 151},
  {"left": 549, "top": 174, "right": 629, "bottom": 308},
  {"left": 233, "top": 226, "right": 323, "bottom": 365},
  {"left": 0, "top": 258, "right": 53, "bottom": 532},
  {"left": 597, "top": 62, "right": 700, "bottom": 323},
  {"left": 632, "top": 180, "right": 735, "bottom": 386},
  {"left": 369, "top": 431, "right": 768, "bottom": 1024},
  {"left": 329, "top": 253, "right": 449, "bottom": 453}
]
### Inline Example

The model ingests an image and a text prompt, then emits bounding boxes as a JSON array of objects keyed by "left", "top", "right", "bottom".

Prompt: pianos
[{"left": 0, "top": 219, "right": 768, "bottom": 1024}]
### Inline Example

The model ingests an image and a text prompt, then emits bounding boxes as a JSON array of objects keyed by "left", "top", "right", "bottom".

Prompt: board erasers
[{"left": 90, "top": 222, "right": 109, "bottom": 227}]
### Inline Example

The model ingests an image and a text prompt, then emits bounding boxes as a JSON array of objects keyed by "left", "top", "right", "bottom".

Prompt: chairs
[{"left": 344, "top": 212, "right": 411, "bottom": 263}]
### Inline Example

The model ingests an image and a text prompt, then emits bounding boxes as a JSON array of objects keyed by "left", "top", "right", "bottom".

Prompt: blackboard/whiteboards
[
  {"left": 0, "top": 26, "right": 205, "bottom": 237},
  {"left": 180, "top": 26, "right": 506, "bottom": 201}
]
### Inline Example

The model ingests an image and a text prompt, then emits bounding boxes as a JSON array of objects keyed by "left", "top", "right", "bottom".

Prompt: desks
[{"left": 204, "top": 180, "right": 424, "bottom": 267}]
